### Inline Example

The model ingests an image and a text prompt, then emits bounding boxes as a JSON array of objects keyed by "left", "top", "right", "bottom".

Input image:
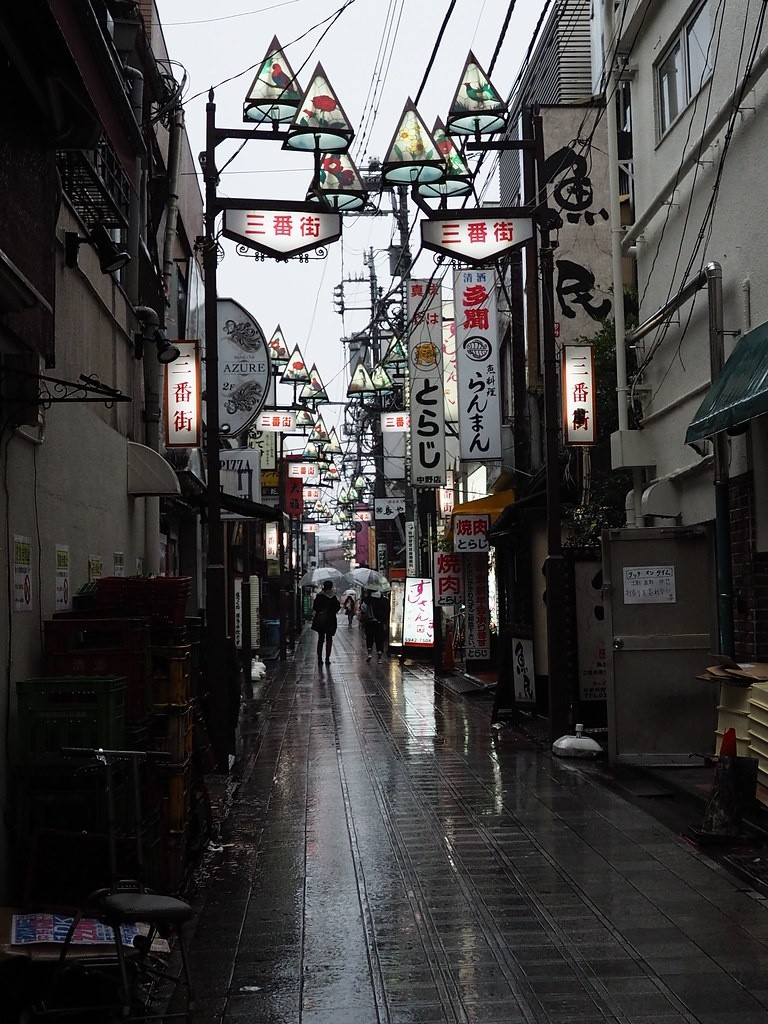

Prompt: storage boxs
[
  {"left": 22, "top": 832, "right": 146, "bottom": 909},
  {"left": 20, "top": 757, "right": 147, "bottom": 837},
  {"left": 155, "top": 822, "right": 191, "bottom": 880},
  {"left": 10, "top": 674, "right": 128, "bottom": 757},
  {"left": 151, "top": 697, "right": 197, "bottom": 761},
  {"left": 148, "top": 753, "right": 195, "bottom": 821},
  {"left": 97, "top": 573, "right": 195, "bottom": 626},
  {"left": 41, "top": 615, "right": 156, "bottom": 722},
  {"left": 154, "top": 644, "right": 196, "bottom": 704}
]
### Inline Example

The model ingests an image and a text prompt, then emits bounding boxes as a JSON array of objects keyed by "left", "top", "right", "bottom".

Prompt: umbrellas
[
  {"left": 343, "top": 589, "right": 358, "bottom": 597},
  {"left": 344, "top": 567, "right": 389, "bottom": 598},
  {"left": 299, "top": 566, "right": 344, "bottom": 597}
]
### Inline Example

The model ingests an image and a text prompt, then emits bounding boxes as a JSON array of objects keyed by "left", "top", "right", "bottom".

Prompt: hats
[{"left": 323, "top": 580, "right": 333, "bottom": 585}]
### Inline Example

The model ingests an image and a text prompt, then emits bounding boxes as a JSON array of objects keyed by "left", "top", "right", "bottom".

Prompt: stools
[{"left": 23, "top": 880, "right": 196, "bottom": 1024}]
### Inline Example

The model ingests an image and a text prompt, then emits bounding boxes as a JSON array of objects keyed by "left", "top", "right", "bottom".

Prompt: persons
[
  {"left": 309, "top": 580, "right": 342, "bottom": 669},
  {"left": 361, "top": 591, "right": 391, "bottom": 664},
  {"left": 344, "top": 596, "right": 356, "bottom": 625}
]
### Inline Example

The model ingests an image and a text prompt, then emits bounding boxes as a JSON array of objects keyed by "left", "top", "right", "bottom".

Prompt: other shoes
[
  {"left": 366, "top": 655, "right": 372, "bottom": 663},
  {"left": 318, "top": 661, "right": 323, "bottom": 667},
  {"left": 325, "top": 661, "right": 331, "bottom": 666},
  {"left": 377, "top": 659, "right": 382, "bottom": 664}
]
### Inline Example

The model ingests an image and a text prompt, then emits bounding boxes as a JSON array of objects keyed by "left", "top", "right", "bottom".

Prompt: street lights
[
  {"left": 257, "top": 317, "right": 396, "bottom": 662},
  {"left": 384, "top": 57, "right": 596, "bottom": 725},
  {"left": 187, "top": 31, "right": 370, "bottom": 772}
]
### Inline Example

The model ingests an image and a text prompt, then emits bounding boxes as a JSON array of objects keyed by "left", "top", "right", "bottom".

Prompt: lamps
[
  {"left": 61, "top": 223, "right": 131, "bottom": 277},
  {"left": 132, "top": 328, "right": 180, "bottom": 366}
]
